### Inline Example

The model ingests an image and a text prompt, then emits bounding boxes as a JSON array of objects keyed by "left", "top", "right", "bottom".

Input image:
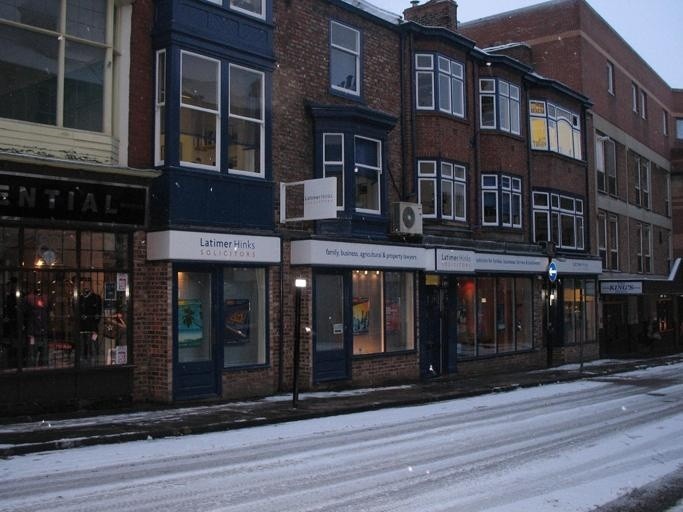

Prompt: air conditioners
[{"left": 390, "top": 202, "right": 423, "bottom": 234}]
